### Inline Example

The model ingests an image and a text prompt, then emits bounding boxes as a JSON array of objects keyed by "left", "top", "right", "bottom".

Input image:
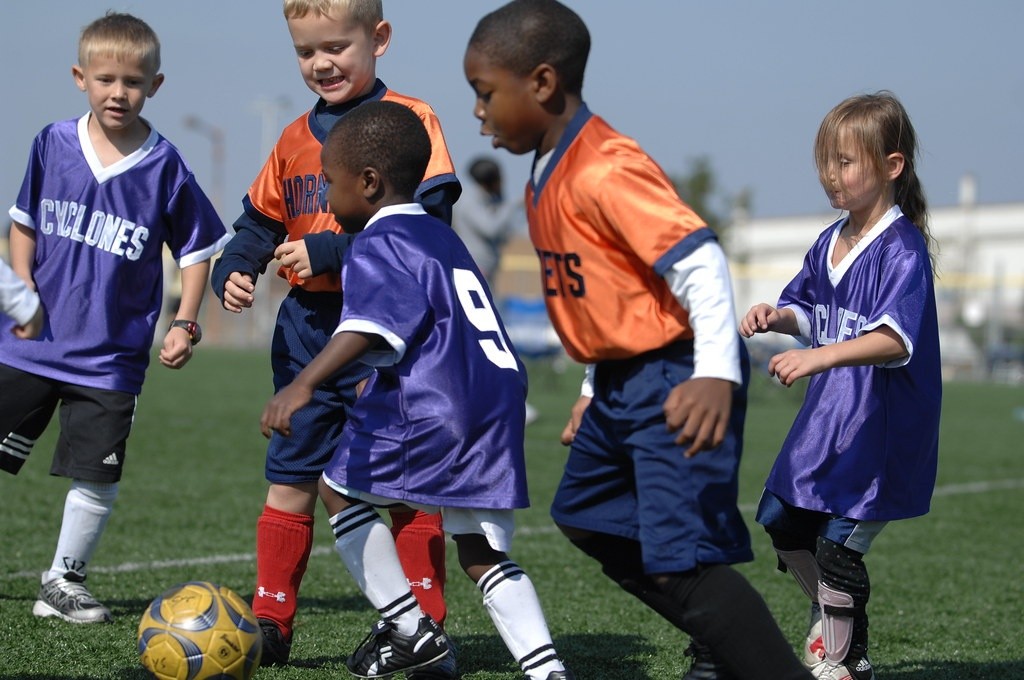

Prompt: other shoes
[
  {"left": 773, "top": 546, "right": 875, "bottom": 680},
  {"left": 680, "top": 641, "right": 718, "bottom": 679}
]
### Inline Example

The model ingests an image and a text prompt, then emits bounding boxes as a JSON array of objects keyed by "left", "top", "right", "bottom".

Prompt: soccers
[{"left": 137, "top": 581, "right": 264, "bottom": 680}]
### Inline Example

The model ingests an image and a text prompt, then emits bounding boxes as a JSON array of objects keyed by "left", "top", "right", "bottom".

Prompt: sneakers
[
  {"left": 407, "top": 633, "right": 456, "bottom": 680},
  {"left": 256, "top": 616, "right": 293, "bottom": 665},
  {"left": 347, "top": 614, "right": 450, "bottom": 680},
  {"left": 32, "top": 571, "right": 111, "bottom": 623}
]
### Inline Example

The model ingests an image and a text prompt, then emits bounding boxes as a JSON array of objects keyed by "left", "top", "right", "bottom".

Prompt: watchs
[{"left": 167, "top": 317, "right": 203, "bottom": 345}]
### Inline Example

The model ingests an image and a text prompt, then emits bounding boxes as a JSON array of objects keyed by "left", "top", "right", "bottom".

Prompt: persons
[
  {"left": 463, "top": 0, "right": 812, "bottom": 680},
  {"left": 1, "top": 7, "right": 235, "bottom": 627},
  {"left": 452, "top": 157, "right": 541, "bottom": 426},
  {"left": 260, "top": 99, "right": 569, "bottom": 679},
  {"left": 735, "top": 87, "right": 945, "bottom": 679},
  {"left": 211, "top": 0, "right": 462, "bottom": 676}
]
